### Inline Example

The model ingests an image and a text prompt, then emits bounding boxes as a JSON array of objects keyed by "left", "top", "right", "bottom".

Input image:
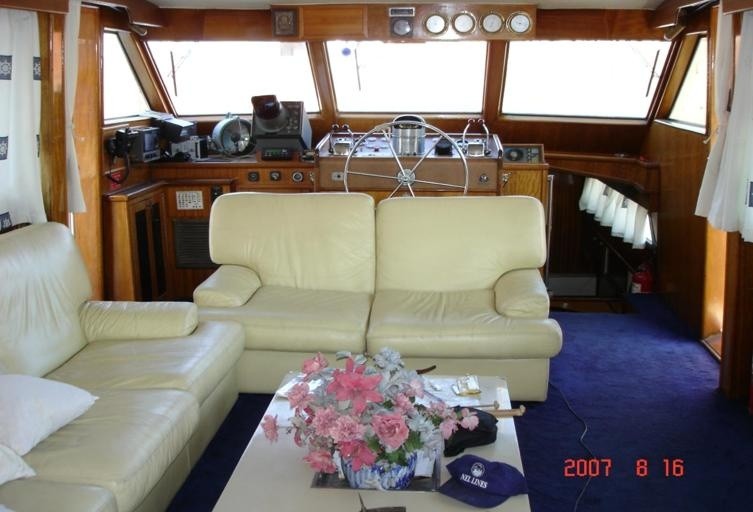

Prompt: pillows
[{"left": 3, "top": 368, "right": 100, "bottom": 486}]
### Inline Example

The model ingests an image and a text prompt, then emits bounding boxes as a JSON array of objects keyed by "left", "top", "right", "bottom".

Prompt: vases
[{"left": 339, "top": 447, "right": 419, "bottom": 493}]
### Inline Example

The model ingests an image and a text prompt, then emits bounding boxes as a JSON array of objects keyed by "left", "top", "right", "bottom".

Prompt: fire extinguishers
[{"left": 631, "top": 260, "right": 653, "bottom": 294}]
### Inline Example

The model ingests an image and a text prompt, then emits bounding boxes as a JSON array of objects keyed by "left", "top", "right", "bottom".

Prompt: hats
[{"left": 438, "top": 455, "right": 529, "bottom": 509}]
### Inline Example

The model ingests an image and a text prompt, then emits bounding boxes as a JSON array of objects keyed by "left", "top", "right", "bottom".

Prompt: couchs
[
  {"left": 192, "top": 190, "right": 565, "bottom": 406},
  {"left": 2, "top": 221, "right": 245, "bottom": 512}
]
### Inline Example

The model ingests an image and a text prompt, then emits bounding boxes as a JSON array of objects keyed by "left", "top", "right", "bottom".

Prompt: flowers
[{"left": 260, "top": 347, "right": 483, "bottom": 483}]
